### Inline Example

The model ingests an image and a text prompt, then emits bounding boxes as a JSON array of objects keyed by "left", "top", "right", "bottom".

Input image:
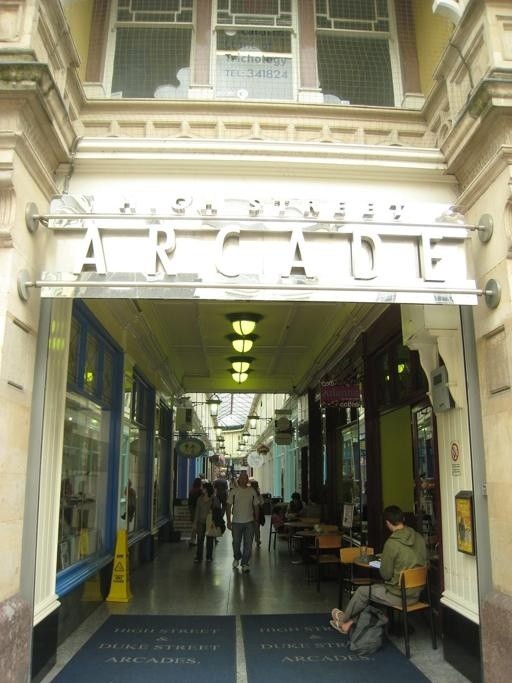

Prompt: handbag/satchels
[{"left": 204, "top": 497, "right": 225, "bottom": 537}]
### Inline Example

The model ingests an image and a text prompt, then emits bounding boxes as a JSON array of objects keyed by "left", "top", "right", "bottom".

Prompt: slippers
[{"left": 329, "top": 607, "right": 348, "bottom": 635}]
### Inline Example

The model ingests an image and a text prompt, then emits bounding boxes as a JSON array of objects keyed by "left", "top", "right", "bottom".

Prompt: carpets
[
  {"left": 52, "top": 609, "right": 241, "bottom": 682},
  {"left": 243, "top": 608, "right": 430, "bottom": 682}
]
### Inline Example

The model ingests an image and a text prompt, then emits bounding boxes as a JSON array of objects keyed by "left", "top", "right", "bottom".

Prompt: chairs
[{"left": 267, "top": 503, "right": 438, "bottom": 659}]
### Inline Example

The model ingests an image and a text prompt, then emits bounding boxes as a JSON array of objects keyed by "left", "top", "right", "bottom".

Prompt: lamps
[
  {"left": 234, "top": 408, "right": 272, "bottom": 458},
  {"left": 190, "top": 393, "right": 226, "bottom": 457},
  {"left": 223, "top": 311, "right": 264, "bottom": 384}
]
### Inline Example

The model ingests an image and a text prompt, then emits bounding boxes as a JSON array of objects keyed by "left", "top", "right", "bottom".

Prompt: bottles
[{"left": 360, "top": 539, "right": 367, "bottom": 563}]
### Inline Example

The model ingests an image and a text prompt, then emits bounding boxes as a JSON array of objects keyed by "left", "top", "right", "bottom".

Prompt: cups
[{"left": 314, "top": 524, "right": 319, "bottom": 532}]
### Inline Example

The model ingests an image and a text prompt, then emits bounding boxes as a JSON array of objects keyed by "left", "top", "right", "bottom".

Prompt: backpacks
[{"left": 349, "top": 606, "right": 389, "bottom": 654}]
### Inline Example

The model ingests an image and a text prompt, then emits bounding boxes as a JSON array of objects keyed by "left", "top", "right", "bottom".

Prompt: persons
[
  {"left": 154, "top": 481, "right": 160, "bottom": 514},
  {"left": 121, "top": 479, "right": 137, "bottom": 523},
  {"left": 188, "top": 471, "right": 321, "bottom": 573},
  {"left": 329, "top": 505, "right": 427, "bottom": 635}
]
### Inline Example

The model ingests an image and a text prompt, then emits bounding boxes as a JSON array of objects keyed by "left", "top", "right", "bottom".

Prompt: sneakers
[
  {"left": 232, "top": 558, "right": 250, "bottom": 572},
  {"left": 193, "top": 556, "right": 213, "bottom": 563}
]
